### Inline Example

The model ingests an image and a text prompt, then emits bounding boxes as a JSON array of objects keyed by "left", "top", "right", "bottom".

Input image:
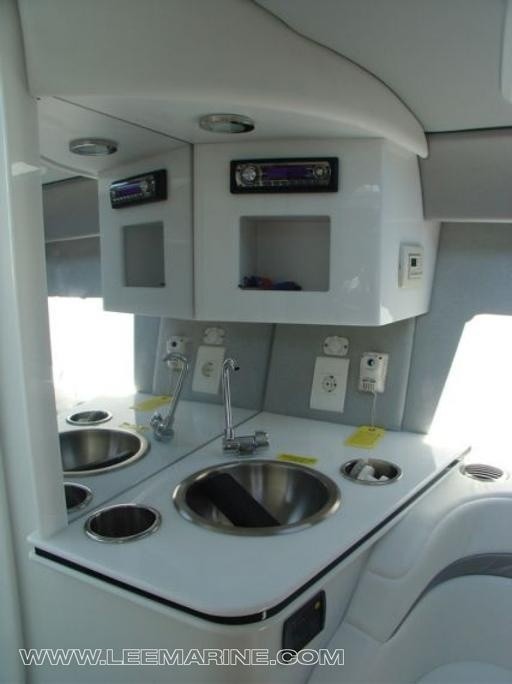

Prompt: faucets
[{"left": 221, "top": 355, "right": 269, "bottom": 455}]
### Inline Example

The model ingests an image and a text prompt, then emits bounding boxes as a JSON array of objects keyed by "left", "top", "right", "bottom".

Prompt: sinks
[
  {"left": 151, "top": 352, "right": 188, "bottom": 443},
  {"left": 59, "top": 428, "right": 151, "bottom": 479},
  {"left": 174, "top": 459, "right": 343, "bottom": 538}
]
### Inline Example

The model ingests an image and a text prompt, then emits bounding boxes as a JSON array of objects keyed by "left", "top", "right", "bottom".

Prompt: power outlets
[
  {"left": 192, "top": 346, "right": 227, "bottom": 395},
  {"left": 310, "top": 356, "right": 350, "bottom": 413}
]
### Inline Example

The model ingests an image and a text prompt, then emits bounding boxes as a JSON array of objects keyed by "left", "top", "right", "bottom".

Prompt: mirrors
[{"left": 35, "top": 96, "right": 277, "bottom": 525}]
